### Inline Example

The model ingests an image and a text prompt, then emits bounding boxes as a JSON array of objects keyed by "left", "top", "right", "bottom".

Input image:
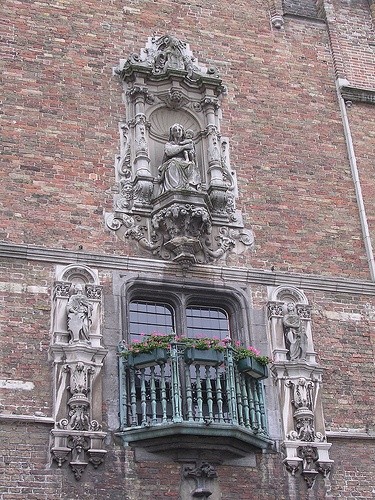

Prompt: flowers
[
  {"left": 118, "top": 331, "right": 179, "bottom": 358},
  {"left": 180, "top": 334, "right": 228, "bottom": 353},
  {"left": 233, "top": 340, "right": 270, "bottom": 366}
]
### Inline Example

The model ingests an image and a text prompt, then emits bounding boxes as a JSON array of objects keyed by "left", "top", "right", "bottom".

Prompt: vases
[
  {"left": 184, "top": 347, "right": 224, "bottom": 368},
  {"left": 238, "top": 356, "right": 268, "bottom": 381},
  {"left": 127, "top": 347, "right": 168, "bottom": 370}
]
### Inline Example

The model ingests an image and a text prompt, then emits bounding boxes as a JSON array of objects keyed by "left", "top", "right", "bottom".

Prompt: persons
[
  {"left": 179, "top": 128, "right": 197, "bottom": 166},
  {"left": 67, "top": 283, "right": 93, "bottom": 346},
  {"left": 155, "top": 123, "right": 202, "bottom": 194},
  {"left": 283, "top": 301, "right": 304, "bottom": 363}
]
